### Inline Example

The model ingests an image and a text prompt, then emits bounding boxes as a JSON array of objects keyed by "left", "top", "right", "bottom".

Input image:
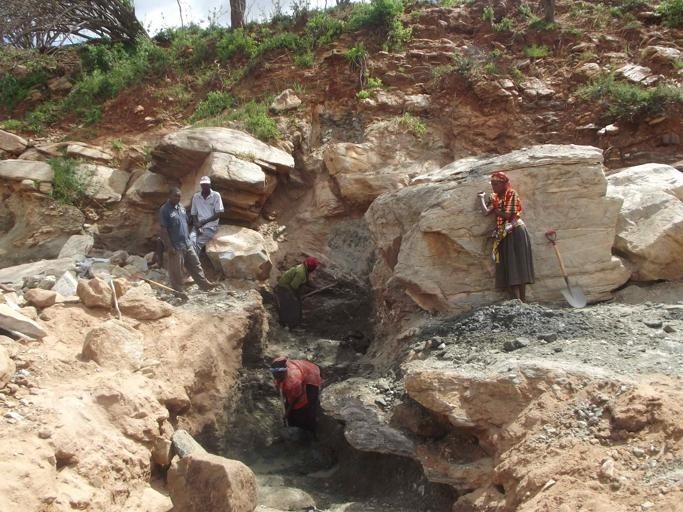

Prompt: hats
[
  {"left": 270, "top": 360, "right": 287, "bottom": 371},
  {"left": 199, "top": 176, "right": 212, "bottom": 185}
]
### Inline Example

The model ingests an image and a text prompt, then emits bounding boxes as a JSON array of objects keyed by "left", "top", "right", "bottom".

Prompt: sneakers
[{"left": 204, "top": 282, "right": 217, "bottom": 290}]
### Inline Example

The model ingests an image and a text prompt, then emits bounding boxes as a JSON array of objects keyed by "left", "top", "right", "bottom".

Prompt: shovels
[
  {"left": 138, "top": 276, "right": 190, "bottom": 300},
  {"left": 545, "top": 231, "right": 587, "bottom": 309}
]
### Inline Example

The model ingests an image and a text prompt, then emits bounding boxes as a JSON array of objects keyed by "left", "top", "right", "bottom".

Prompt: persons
[
  {"left": 270, "top": 354, "right": 321, "bottom": 445},
  {"left": 475, "top": 171, "right": 535, "bottom": 305},
  {"left": 157, "top": 185, "right": 218, "bottom": 301},
  {"left": 182, "top": 175, "right": 225, "bottom": 282},
  {"left": 276, "top": 256, "right": 320, "bottom": 327}
]
